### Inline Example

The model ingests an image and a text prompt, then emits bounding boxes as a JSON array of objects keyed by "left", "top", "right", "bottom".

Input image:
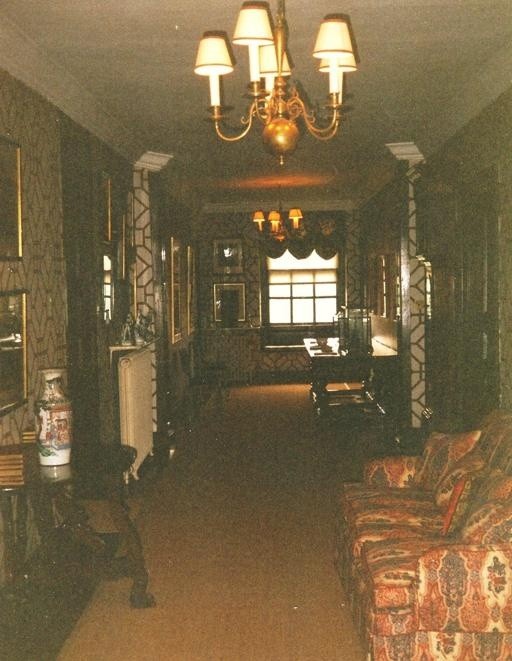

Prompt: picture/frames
[
  {"left": 1, "top": 134, "right": 26, "bottom": 261},
  {"left": 212, "top": 238, "right": 244, "bottom": 275},
  {"left": 214, "top": 282, "right": 246, "bottom": 323},
  {"left": 1, "top": 291, "right": 31, "bottom": 417}
]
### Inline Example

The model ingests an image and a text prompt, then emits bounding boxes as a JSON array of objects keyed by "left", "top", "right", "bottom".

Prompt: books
[
  {"left": 21, "top": 429, "right": 39, "bottom": 444},
  {"left": 0, "top": 444, "right": 27, "bottom": 488}
]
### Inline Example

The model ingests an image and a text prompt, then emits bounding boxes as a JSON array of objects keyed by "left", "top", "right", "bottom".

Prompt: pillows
[{"left": 416, "top": 429, "right": 512, "bottom": 545}]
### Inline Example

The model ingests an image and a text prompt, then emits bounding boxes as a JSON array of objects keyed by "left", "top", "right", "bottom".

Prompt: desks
[{"left": 0, "top": 441, "right": 152, "bottom": 618}]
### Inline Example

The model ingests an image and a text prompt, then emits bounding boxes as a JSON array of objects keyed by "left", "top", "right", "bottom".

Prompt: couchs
[{"left": 330, "top": 409, "right": 512, "bottom": 660}]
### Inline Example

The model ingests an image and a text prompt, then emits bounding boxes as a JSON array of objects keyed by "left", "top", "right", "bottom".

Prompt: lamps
[
  {"left": 193, "top": 1, "right": 359, "bottom": 172},
  {"left": 251, "top": 181, "right": 304, "bottom": 244}
]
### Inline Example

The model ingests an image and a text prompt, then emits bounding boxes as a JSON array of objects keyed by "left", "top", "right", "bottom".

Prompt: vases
[{"left": 33, "top": 368, "right": 75, "bottom": 467}]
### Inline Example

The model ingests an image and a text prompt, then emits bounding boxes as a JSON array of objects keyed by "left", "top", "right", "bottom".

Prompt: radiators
[{"left": 117, "top": 347, "right": 155, "bottom": 484}]
